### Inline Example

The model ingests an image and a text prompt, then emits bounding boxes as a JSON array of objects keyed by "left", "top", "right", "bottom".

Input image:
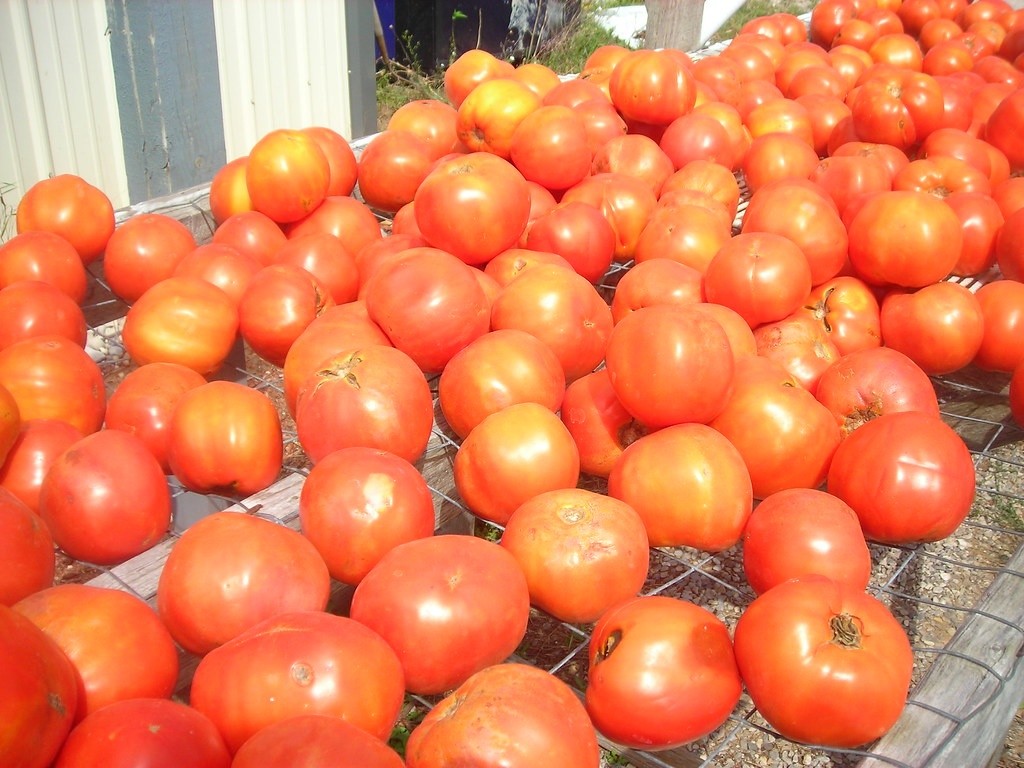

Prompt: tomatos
[{"left": 0, "top": 0, "right": 1024, "bottom": 768}]
